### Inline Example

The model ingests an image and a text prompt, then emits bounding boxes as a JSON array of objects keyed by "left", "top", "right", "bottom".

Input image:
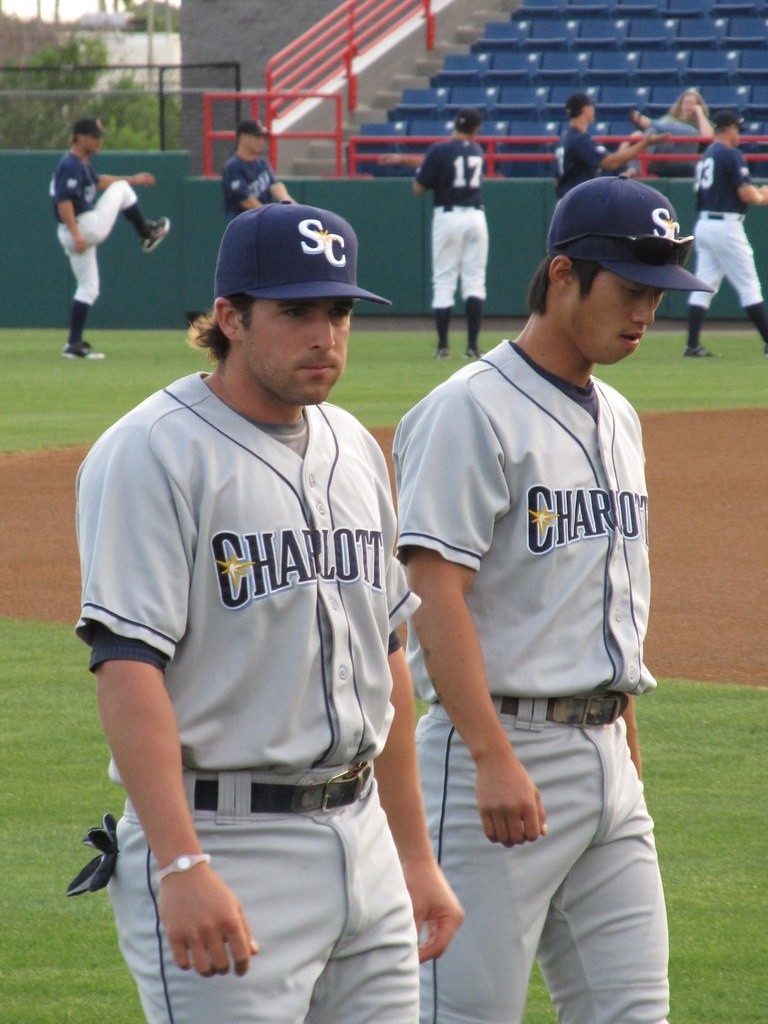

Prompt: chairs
[{"left": 345, "top": 0, "right": 768, "bottom": 179}]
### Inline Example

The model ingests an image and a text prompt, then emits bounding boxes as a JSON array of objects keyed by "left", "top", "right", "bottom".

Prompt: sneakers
[
  {"left": 465, "top": 348, "right": 485, "bottom": 360},
  {"left": 433, "top": 347, "right": 449, "bottom": 359},
  {"left": 140, "top": 218, "right": 170, "bottom": 253},
  {"left": 684, "top": 345, "right": 719, "bottom": 358},
  {"left": 62, "top": 341, "right": 105, "bottom": 360}
]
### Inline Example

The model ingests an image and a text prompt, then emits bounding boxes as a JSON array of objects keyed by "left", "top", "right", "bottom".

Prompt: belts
[
  {"left": 195, "top": 768, "right": 372, "bottom": 812},
  {"left": 500, "top": 694, "right": 627, "bottom": 725},
  {"left": 708, "top": 212, "right": 742, "bottom": 221},
  {"left": 443, "top": 203, "right": 484, "bottom": 211}
]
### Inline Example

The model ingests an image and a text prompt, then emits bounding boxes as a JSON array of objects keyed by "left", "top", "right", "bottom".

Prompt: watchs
[{"left": 156, "top": 853, "right": 212, "bottom": 885}]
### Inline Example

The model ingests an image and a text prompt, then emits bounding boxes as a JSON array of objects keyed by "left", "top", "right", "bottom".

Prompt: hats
[
  {"left": 73, "top": 118, "right": 107, "bottom": 138},
  {"left": 213, "top": 201, "right": 393, "bottom": 307},
  {"left": 454, "top": 106, "right": 484, "bottom": 124},
  {"left": 711, "top": 110, "right": 745, "bottom": 129},
  {"left": 565, "top": 92, "right": 598, "bottom": 113},
  {"left": 547, "top": 171, "right": 715, "bottom": 294},
  {"left": 236, "top": 119, "right": 270, "bottom": 136}
]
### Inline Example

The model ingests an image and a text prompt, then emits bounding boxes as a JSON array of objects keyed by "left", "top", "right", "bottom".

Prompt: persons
[
  {"left": 393, "top": 173, "right": 715, "bottom": 1024},
  {"left": 49, "top": 116, "right": 169, "bottom": 359},
  {"left": 412, "top": 109, "right": 489, "bottom": 358},
  {"left": 224, "top": 120, "right": 300, "bottom": 220},
  {"left": 685, "top": 111, "right": 768, "bottom": 358},
  {"left": 554, "top": 88, "right": 714, "bottom": 199},
  {"left": 76, "top": 202, "right": 466, "bottom": 1024}
]
